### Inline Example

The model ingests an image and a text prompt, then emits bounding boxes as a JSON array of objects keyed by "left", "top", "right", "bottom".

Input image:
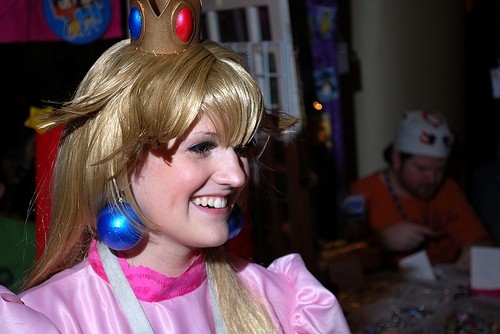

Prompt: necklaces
[{"left": 384, "top": 167, "right": 429, "bottom": 227}]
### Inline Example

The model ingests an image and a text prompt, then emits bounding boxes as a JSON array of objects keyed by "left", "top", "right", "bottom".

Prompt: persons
[
  {"left": 340, "top": 109, "right": 488, "bottom": 272},
  {"left": 0, "top": 39, "right": 352, "bottom": 334}
]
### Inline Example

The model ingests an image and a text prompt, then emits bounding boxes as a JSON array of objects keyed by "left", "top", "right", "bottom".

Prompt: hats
[{"left": 395, "top": 110, "right": 453, "bottom": 159}]
